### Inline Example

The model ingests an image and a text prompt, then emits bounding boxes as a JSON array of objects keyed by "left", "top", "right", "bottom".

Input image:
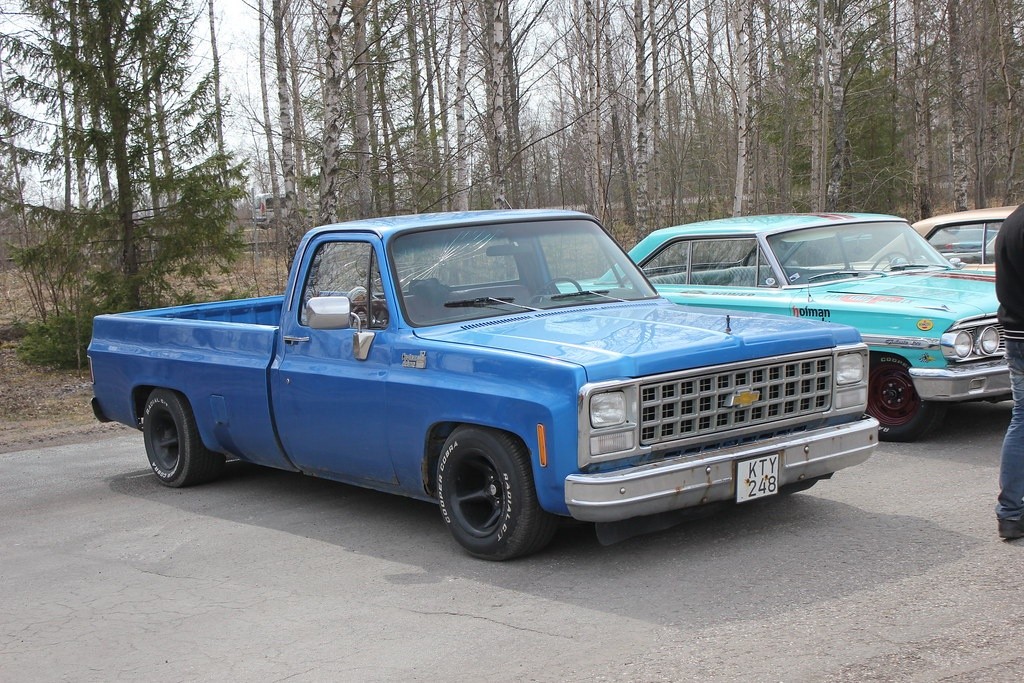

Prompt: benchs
[
  {"left": 405, "top": 283, "right": 532, "bottom": 328},
  {"left": 650, "top": 266, "right": 774, "bottom": 288}
]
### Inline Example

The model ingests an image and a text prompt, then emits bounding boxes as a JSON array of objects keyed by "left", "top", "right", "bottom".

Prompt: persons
[{"left": 995, "top": 204, "right": 1024, "bottom": 540}]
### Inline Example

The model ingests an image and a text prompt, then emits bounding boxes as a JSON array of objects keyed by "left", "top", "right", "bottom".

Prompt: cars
[
  {"left": 555, "top": 213, "right": 1014, "bottom": 442},
  {"left": 809, "top": 206, "right": 1016, "bottom": 271}
]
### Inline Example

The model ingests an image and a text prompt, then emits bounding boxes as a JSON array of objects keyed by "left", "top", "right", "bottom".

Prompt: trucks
[{"left": 252, "top": 194, "right": 323, "bottom": 228}]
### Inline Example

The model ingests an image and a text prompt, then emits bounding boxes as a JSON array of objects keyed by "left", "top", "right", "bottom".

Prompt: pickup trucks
[{"left": 86, "top": 210, "right": 878, "bottom": 562}]
